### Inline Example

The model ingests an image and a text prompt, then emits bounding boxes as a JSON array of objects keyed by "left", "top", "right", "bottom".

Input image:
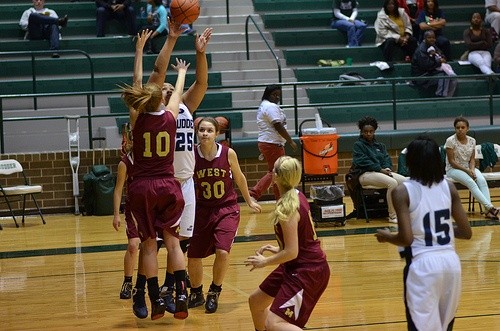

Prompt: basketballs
[{"left": 170, "top": 0, "right": 200, "bottom": 24}]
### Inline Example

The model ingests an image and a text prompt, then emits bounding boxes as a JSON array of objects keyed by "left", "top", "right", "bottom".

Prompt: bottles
[
  {"left": 140, "top": 6, "right": 145, "bottom": 18},
  {"left": 429, "top": 15, "right": 433, "bottom": 23}
]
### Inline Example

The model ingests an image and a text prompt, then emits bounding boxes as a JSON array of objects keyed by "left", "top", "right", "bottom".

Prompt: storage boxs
[{"left": 308, "top": 201, "right": 346, "bottom": 221}]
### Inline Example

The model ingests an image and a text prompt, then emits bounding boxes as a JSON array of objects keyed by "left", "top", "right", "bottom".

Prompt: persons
[
  {"left": 444, "top": 117, "right": 500, "bottom": 219},
  {"left": 352, "top": 116, "right": 409, "bottom": 224},
  {"left": 19, "top": 0, "right": 68, "bottom": 59},
  {"left": 248, "top": 85, "right": 297, "bottom": 203},
  {"left": 374, "top": 135, "right": 472, "bottom": 331},
  {"left": 95, "top": 0, "right": 262, "bottom": 320},
  {"left": 244, "top": 156, "right": 330, "bottom": 331},
  {"left": 330, "top": 0, "right": 500, "bottom": 98}
]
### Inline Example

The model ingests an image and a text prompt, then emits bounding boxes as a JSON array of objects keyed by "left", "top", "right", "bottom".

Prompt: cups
[
  {"left": 314, "top": 113, "right": 322, "bottom": 130},
  {"left": 45, "top": 11, "right": 50, "bottom": 16},
  {"left": 347, "top": 58, "right": 353, "bottom": 66}
]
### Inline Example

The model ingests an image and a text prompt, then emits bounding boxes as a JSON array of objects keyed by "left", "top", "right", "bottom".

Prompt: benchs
[
  {"left": 0, "top": 0, "right": 244, "bottom": 152},
  {"left": 252, "top": 0, "right": 500, "bottom": 124}
]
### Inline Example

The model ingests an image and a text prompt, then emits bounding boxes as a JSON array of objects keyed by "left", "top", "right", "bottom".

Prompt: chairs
[
  {"left": 440, "top": 142, "right": 500, "bottom": 214},
  {"left": 345, "top": 170, "right": 388, "bottom": 223},
  {"left": 0, "top": 158, "right": 46, "bottom": 229}
]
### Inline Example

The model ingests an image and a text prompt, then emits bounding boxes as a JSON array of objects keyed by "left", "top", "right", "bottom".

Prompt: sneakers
[
  {"left": 174, "top": 295, "right": 188, "bottom": 319},
  {"left": 159, "top": 285, "right": 175, "bottom": 314},
  {"left": 204, "top": 287, "right": 221, "bottom": 312},
  {"left": 119, "top": 282, "right": 132, "bottom": 299},
  {"left": 132, "top": 288, "right": 148, "bottom": 318},
  {"left": 187, "top": 290, "right": 205, "bottom": 308},
  {"left": 150, "top": 297, "right": 165, "bottom": 319}
]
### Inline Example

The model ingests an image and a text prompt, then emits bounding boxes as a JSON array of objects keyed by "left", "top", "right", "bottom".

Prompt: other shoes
[
  {"left": 389, "top": 217, "right": 398, "bottom": 224},
  {"left": 184, "top": 29, "right": 196, "bottom": 34},
  {"left": 60, "top": 15, "right": 67, "bottom": 27},
  {"left": 52, "top": 53, "right": 59, "bottom": 58}
]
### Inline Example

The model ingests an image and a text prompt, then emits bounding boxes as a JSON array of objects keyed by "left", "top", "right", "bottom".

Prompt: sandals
[
  {"left": 485, "top": 211, "right": 497, "bottom": 218},
  {"left": 491, "top": 207, "right": 499, "bottom": 215}
]
